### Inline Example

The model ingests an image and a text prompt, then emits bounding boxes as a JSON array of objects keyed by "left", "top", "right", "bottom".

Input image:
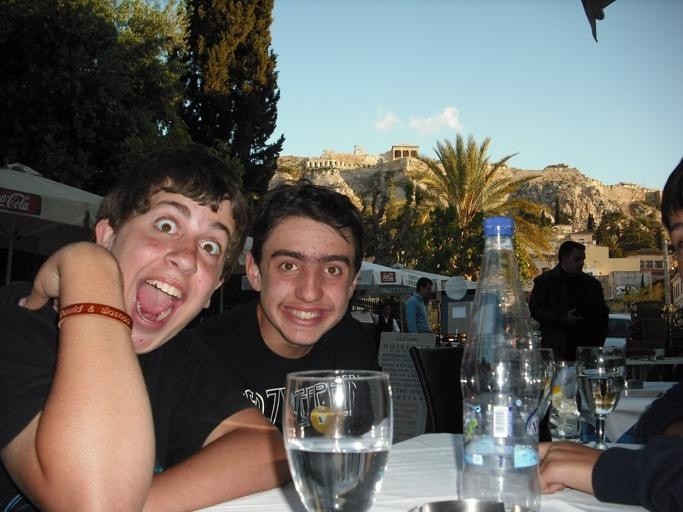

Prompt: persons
[
  {"left": 0, "top": 147, "right": 291, "bottom": 512},
  {"left": 527, "top": 241, "right": 610, "bottom": 359},
  {"left": 518, "top": 155, "right": 683, "bottom": 512},
  {"left": 371, "top": 304, "right": 402, "bottom": 352},
  {"left": 192, "top": 178, "right": 380, "bottom": 441},
  {"left": 404, "top": 277, "right": 434, "bottom": 334}
]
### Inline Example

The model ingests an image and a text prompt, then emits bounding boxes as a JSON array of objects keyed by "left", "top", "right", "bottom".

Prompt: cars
[{"left": 347, "top": 307, "right": 400, "bottom": 346}]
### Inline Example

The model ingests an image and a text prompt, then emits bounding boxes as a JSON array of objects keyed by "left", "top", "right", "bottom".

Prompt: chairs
[
  {"left": 624, "top": 301, "right": 682, "bottom": 382},
  {"left": 407, "top": 346, "right": 463, "bottom": 434}
]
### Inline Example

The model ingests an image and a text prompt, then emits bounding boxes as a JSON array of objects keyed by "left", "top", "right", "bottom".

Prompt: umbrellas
[
  {"left": 220, "top": 236, "right": 255, "bottom": 317},
  {"left": 356, "top": 261, "right": 422, "bottom": 289},
  {"left": 0, "top": 159, "right": 104, "bottom": 284},
  {"left": 356, "top": 262, "right": 478, "bottom": 333}
]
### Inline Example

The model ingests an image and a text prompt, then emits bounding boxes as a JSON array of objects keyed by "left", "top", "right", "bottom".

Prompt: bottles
[{"left": 460, "top": 217, "right": 542, "bottom": 512}]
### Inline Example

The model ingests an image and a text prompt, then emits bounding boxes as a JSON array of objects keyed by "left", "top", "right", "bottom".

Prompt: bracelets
[{"left": 57, "top": 301, "right": 135, "bottom": 331}]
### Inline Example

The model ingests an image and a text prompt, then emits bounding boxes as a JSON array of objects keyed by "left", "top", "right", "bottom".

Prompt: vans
[{"left": 601, "top": 313, "right": 633, "bottom": 367}]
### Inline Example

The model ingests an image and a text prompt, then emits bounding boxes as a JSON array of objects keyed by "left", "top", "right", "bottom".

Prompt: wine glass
[{"left": 576, "top": 346, "right": 626, "bottom": 450}]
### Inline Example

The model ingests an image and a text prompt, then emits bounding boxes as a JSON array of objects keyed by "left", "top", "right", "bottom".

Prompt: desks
[{"left": 192, "top": 432, "right": 650, "bottom": 511}]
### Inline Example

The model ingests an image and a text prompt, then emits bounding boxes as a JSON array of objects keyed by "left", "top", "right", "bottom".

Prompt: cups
[
  {"left": 541, "top": 348, "right": 578, "bottom": 405},
  {"left": 282, "top": 371, "right": 393, "bottom": 512}
]
